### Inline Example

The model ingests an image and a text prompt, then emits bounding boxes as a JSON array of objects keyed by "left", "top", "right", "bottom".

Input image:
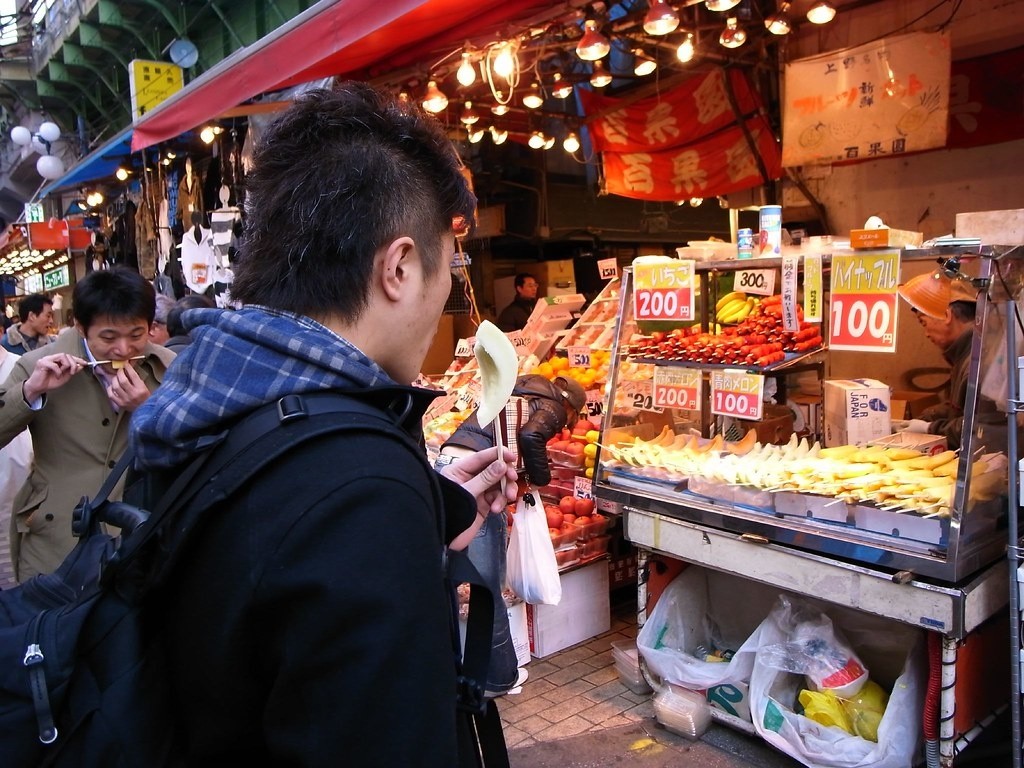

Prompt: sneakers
[{"left": 484, "top": 668, "right": 528, "bottom": 698}]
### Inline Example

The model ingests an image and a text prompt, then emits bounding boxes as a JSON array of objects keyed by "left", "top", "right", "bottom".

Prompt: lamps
[
  {"left": 421, "top": 0, "right": 837, "bottom": 208},
  {"left": 898, "top": 256, "right": 994, "bottom": 322}
]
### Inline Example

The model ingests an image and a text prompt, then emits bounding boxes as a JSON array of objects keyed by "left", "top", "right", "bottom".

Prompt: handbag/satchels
[{"left": 505, "top": 488, "right": 562, "bottom": 607}]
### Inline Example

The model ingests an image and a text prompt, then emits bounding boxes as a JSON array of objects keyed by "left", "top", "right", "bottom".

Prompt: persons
[
  {"left": 499, "top": 272, "right": 539, "bottom": 333},
  {"left": 117, "top": 81, "right": 518, "bottom": 768},
  {"left": 433, "top": 372, "right": 587, "bottom": 699},
  {"left": 1, "top": 268, "right": 214, "bottom": 591},
  {"left": 892, "top": 295, "right": 1024, "bottom": 462}
]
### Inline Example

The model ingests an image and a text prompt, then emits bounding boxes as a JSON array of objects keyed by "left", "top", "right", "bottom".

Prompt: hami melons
[
  {"left": 609, "top": 425, "right": 820, "bottom": 489},
  {"left": 764, "top": 444, "right": 993, "bottom": 516}
]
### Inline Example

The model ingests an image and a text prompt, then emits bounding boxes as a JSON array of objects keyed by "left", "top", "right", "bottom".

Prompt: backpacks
[{"left": 0, "top": 395, "right": 448, "bottom": 768}]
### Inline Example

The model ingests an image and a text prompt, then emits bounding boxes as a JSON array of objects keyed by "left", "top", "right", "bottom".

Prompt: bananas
[{"left": 692, "top": 292, "right": 760, "bottom": 334}]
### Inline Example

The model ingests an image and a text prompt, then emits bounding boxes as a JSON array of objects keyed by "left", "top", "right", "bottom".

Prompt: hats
[{"left": 553, "top": 376, "right": 587, "bottom": 424}]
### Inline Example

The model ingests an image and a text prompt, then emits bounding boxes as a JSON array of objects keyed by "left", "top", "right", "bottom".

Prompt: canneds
[
  {"left": 737, "top": 228, "right": 753, "bottom": 259},
  {"left": 758, "top": 204, "right": 782, "bottom": 258}
]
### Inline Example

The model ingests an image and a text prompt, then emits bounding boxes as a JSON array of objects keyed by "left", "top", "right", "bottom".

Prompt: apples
[{"left": 498, "top": 419, "right": 607, "bottom": 564}]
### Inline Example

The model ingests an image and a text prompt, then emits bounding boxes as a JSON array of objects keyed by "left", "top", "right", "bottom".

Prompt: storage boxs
[{"left": 409, "top": 241, "right": 1012, "bottom": 768}]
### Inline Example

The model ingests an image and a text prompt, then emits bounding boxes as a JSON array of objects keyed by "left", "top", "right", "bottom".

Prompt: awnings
[
  {"left": 37, "top": 127, "right": 151, "bottom": 206},
  {"left": 0, "top": 224, "right": 28, "bottom": 249},
  {"left": 128, "top": 0, "right": 577, "bottom": 155}
]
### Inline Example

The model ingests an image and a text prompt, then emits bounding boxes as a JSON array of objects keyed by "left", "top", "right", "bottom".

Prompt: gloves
[{"left": 896, "top": 419, "right": 933, "bottom": 434}]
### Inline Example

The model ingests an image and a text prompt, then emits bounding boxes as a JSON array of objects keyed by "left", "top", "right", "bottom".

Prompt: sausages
[{"left": 630, "top": 296, "right": 822, "bottom": 365}]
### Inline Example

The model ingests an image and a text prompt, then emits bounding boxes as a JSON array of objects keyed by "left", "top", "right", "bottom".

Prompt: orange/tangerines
[
  {"left": 423, "top": 410, "right": 472, "bottom": 443},
  {"left": 529, "top": 350, "right": 611, "bottom": 387}
]
